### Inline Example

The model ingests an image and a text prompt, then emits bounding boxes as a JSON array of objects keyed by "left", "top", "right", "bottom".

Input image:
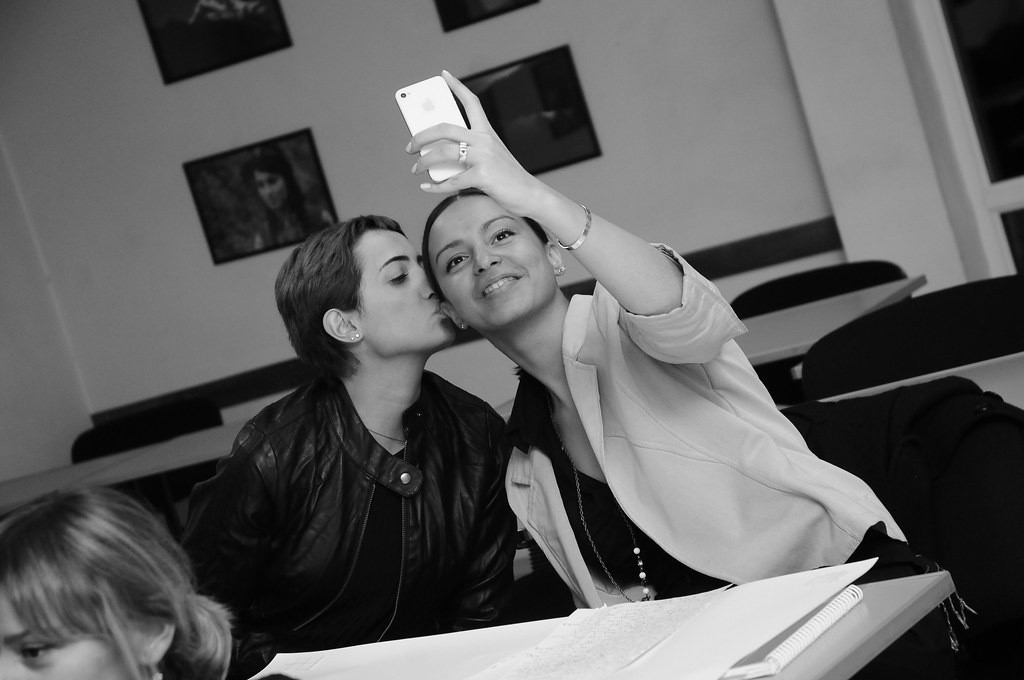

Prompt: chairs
[{"left": 728, "top": 259, "right": 1024, "bottom": 680}]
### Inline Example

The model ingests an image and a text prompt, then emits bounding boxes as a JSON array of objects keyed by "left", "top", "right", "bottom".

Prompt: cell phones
[{"left": 395, "top": 75, "right": 473, "bottom": 183}]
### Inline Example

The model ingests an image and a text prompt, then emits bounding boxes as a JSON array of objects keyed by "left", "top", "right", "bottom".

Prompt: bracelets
[{"left": 556, "top": 205, "right": 591, "bottom": 251}]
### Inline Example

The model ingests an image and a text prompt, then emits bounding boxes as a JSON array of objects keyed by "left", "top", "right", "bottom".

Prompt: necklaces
[
  {"left": 550, "top": 402, "right": 649, "bottom": 603},
  {"left": 368, "top": 428, "right": 406, "bottom": 445}
]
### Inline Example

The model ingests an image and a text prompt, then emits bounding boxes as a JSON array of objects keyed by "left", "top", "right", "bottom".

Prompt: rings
[{"left": 458, "top": 142, "right": 467, "bottom": 165}]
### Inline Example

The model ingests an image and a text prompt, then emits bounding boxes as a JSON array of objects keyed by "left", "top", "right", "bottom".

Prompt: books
[{"left": 717, "top": 584, "right": 864, "bottom": 680}]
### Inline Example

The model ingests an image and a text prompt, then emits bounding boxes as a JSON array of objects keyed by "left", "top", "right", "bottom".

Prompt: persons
[
  {"left": 176, "top": 213, "right": 516, "bottom": 680},
  {"left": 407, "top": 71, "right": 959, "bottom": 680},
  {"left": 242, "top": 147, "right": 334, "bottom": 250},
  {"left": 0, "top": 484, "right": 234, "bottom": 680}
]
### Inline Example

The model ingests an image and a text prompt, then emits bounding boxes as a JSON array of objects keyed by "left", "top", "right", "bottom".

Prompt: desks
[
  {"left": 275, "top": 571, "right": 957, "bottom": 680},
  {"left": 0, "top": 273, "right": 1024, "bottom": 490}
]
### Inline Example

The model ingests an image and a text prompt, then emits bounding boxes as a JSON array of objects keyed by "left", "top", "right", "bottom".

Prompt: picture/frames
[
  {"left": 433, "top": 0, "right": 541, "bottom": 32},
  {"left": 180, "top": 128, "right": 340, "bottom": 265},
  {"left": 135, "top": 0, "right": 295, "bottom": 85},
  {"left": 453, "top": 43, "right": 603, "bottom": 190}
]
